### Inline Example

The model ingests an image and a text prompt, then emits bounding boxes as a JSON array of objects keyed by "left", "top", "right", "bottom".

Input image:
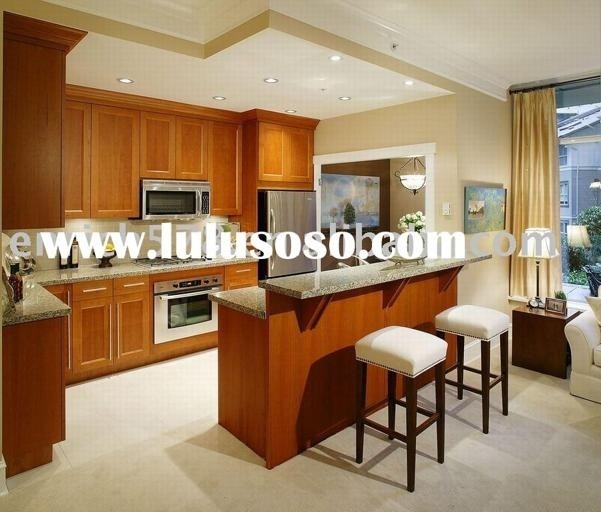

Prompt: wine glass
[{"left": 93, "top": 251, "right": 113, "bottom": 268}]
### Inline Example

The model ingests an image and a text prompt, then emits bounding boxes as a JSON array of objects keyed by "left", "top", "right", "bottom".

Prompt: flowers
[{"left": 397, "top": 209, "right": 427, "bottom": 233}]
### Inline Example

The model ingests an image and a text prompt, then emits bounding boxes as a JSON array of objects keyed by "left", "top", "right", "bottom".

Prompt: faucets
[{"left": 336, "top": 256, "right": 370, "bottom": 269}]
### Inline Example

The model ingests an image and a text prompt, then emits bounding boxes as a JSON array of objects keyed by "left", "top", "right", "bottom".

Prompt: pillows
[{"left": 583, "top": 292, "right": 601, "bottom": 326}]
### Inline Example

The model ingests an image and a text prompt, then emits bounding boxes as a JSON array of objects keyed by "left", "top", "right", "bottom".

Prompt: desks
[
  {"left": 206, "top": 252, "right": 494, "bottom": 471},
  {"left": 512, "top": 304, "right": 583, "bottom": 380}
]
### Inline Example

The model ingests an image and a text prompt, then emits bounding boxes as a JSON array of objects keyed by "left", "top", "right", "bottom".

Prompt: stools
[
  {"left": 434, "top": 302, "right": 512, "bottom": 434},
  {"left": 355, "top": 324, "right": 450, "bottom": 493}
]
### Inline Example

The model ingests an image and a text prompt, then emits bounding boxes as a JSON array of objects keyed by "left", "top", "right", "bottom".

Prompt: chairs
[{"left": 564, "top": 283, "right": 601, "bottom": 405}]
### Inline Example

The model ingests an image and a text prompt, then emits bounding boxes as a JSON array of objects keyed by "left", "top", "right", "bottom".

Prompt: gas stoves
[{"left": 135, "top": 254, "right": 211, "bottom": 268}]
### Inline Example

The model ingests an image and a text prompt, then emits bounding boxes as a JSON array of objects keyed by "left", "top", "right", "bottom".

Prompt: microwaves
[{"left": 140, "top": 180, "right": 212, "bottom": 220}]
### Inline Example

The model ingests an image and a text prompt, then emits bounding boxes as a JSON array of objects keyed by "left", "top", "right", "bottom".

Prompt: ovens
[{"left": 154, "top": 286, "right": 220, "bottom": 345}]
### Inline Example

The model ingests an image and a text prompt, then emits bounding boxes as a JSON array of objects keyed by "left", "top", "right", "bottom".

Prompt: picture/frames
[{"left": 545, "top": 294, "right": 569, "bottom": 316}]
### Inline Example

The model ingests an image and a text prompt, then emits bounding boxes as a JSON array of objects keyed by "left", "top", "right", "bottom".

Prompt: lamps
[
  {"left": 517, "top": 226, "right": 562, "bottom": 310},
  {"left": 393, "top": 157, "right": 426, "bottom": 196}
]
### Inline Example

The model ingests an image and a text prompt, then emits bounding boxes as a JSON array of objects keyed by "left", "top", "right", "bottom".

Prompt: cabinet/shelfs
[
  {"left": 1, "top": 289, "right": 72, "bottom": 479},
  {"left": 64, "top": 79, "right": 321, "bottom": 217},
  {"left": 2, "top": 10, "right": 90, "bottom": 234},
  {"left": 44, "top": 262, "right": 260, "bottom": 387}
]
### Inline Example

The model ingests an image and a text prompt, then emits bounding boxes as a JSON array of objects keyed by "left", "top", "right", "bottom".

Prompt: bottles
[
  {"left": 8, "top": 261, "right": 24, "bottom": 303},
  {"left": 59, "top": 243, "right": 68, "bottom": 269},
  {"left": 70, "top": 236, "right": 79, "bottom": 268}
]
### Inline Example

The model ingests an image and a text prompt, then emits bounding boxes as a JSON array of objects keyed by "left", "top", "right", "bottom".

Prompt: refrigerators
[{"left": 258, "top": 190, "right": 317, "bottom": 282}]
